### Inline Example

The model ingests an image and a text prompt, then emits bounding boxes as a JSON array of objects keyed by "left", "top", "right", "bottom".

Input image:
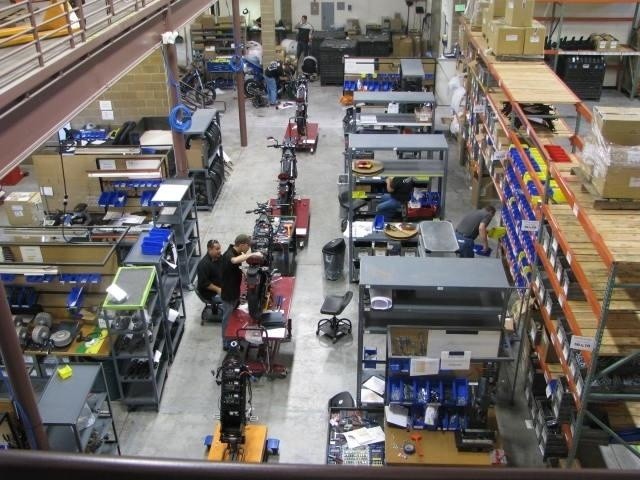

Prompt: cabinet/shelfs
[
  {"left": 1, "top": 110, "right": 225, "bottom": 459},
  {"left": 325, "top": 18, "right": 640, "bottom": 470}
]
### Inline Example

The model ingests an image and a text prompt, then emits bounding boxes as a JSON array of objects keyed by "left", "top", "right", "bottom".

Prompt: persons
[
  {"left": 222, "top": 235, "right": 264, "bottom": 350},
  {"left": 256, "top": 17, "right": 261, "bottom": 28},
  {"left": 294, "top": 15, "right": 313, "bottom": 60},
  {"left": 265, "top": 61, "right": 289, "bottom": 105},
  {"left": 455, "top": 206, "right": 496, "bottom": 258},
  {"left": 376, "top": 177, "right": 414, "bottom": 222},
  {"left": 197, "top": 240, "right": 224, "bottom": 303},
  {"left": 301, "top": 55, "right": 318, "bottom": 79}
]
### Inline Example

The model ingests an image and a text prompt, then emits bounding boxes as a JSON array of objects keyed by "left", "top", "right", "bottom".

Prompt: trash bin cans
[{"left": 322, "top": 238, "right": 346, "bottom": 281}]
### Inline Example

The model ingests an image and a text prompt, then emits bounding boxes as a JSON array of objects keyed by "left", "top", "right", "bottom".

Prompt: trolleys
[{"left": 0, "top": 0, "right": 81, "bottom": 48}]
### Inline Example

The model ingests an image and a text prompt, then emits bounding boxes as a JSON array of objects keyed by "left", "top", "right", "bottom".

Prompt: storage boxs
[
  {"left": 191, "top": 15, "right": 246, "bottom": 83},
  {"left": 479, "top": 0, "right": 546, "bottom": 62}
]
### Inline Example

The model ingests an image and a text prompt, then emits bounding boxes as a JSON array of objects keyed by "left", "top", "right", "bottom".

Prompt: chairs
[{"left": 317, "top": 292, "right": 353, "bottom": 343}]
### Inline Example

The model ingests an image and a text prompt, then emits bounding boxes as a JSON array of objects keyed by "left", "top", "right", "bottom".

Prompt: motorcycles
[
  {"left": 287, "top": 75, "right": 313, "bottom": 136},
  {"left": 266, "top": 135, "right": 312, "bottom": 217},
  {"left": 244, "top": 56, "right": 309, "bottom": 99},
  {"left": 209, "top": 331, "right": 260, "bottom": 465},
  {"left": 244, "top": 200, "right": 284, "bottom": 322},
  {"left": 177, "top": 60, "right": 217, "bottom": 105}
]
